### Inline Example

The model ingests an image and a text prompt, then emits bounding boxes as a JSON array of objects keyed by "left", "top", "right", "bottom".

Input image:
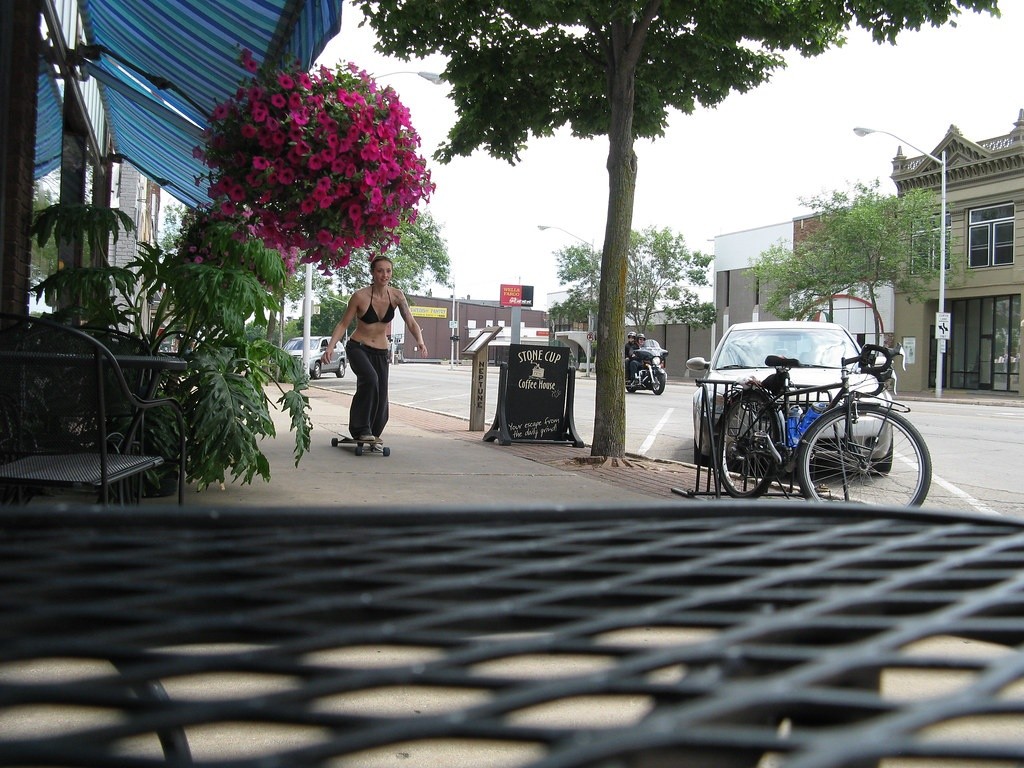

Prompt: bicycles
[{"left": 712, "top": 342, "right": 933, "bottom": 508}]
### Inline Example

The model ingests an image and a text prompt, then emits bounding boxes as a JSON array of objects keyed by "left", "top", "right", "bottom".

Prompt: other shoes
[{"left": 358, "top": 434, "right": 376, "bottom": 441}]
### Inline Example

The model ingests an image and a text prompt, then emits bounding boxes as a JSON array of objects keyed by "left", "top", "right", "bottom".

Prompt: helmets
[
  {"left": 637, "top": 333, "right": 646, "bottom": 339},
  {"left": 627, "top": 331, "right": 638, "bottom": 338}
]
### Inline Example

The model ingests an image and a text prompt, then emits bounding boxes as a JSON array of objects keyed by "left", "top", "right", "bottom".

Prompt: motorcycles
[{"left": 624, "top": 331, "right": 669, "bottom": 396}]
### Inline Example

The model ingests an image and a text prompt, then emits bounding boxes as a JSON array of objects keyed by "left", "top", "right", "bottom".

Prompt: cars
[{"left": 685, "top": 320, "right": 894, "bottom": 477}]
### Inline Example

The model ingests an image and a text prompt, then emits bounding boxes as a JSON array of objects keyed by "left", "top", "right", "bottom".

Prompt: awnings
[
  {"left": 78, "top": 0, "right": 344, "bottom": 219},
  {"left": 33, "top": 37, "right": 64, "bottom": 180}
]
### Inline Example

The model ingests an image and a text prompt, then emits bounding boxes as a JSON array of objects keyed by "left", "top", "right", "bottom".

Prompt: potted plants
[{"left": 25, "top": 203, "right": 313, "bottom": 499}]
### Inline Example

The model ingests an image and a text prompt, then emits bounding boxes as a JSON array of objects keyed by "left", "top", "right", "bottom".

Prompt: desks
[{"left": 1, "top": 350, "right": 190, "bottom": 510}]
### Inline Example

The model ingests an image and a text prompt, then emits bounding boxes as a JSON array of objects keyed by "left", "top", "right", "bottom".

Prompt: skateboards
[{"left": 331, "top": 430, "right": 391, "bottom": 456}]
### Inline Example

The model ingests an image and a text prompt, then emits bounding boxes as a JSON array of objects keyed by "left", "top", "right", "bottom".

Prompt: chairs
[
  {"left": 0, "top": 325, "right": 154, "bottom": 507},
  {"left": 0, "top": 313, "right": 186, "bottom": 506}
]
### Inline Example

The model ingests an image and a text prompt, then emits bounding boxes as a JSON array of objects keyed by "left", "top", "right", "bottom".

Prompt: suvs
[{"left": 281, "top": 336, "right": 347, "bottom": 379}]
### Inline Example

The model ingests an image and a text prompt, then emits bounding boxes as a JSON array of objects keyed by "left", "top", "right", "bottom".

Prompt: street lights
[
  {"left": 319, "top": 295, "right": 349, "bottom": 352},
  {"left": 851, "top": 125, "right": 952, "bottom": 396},
  {"left": 537, "top": 226, "right": 594, "bottom": 378}
]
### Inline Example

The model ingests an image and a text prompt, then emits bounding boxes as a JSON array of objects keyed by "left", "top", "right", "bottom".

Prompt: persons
[
  {"left": 624, "top": 331, "right": 637, "bottom": 384},
  {"left": 321, "top": 256, "right": 429, "bottom": 444},
  {"left": 629, "top": 333, "right": 646, "bottom": 384}
]
